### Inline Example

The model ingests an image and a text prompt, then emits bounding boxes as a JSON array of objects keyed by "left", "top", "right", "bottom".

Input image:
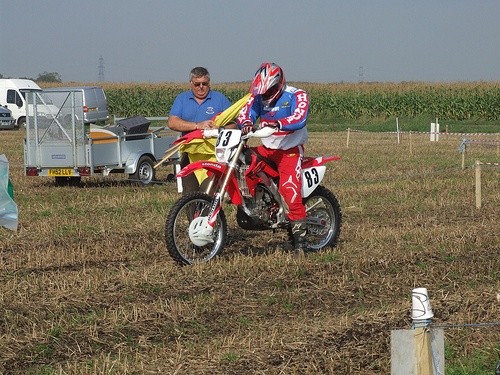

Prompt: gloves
[
  {"left": 258, "top": 120, "right": 277, "bottom": 129},
  {"left": 241, "top": 119, "right": 256, "bottom": 135}
]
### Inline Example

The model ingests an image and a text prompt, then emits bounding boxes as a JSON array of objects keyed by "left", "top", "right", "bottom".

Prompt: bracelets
[{"left": 196, "top": 123, "right": 197, "bottom": 130}]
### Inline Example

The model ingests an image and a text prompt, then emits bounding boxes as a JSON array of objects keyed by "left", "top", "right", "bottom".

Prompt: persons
[
  {"left": 237, "top": 62, "right": 308, "bottom": 254},
  {"left": 169, "top": 67, "right": 238, "bottom": 224}
]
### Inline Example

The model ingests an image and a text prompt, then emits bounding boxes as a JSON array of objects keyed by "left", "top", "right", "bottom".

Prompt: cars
[{"left": 0, "top": 105, "right": 17, "bottom": 130}]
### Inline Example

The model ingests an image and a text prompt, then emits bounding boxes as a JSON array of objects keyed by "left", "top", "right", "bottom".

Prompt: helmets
[{"left": 248, "top": 63, "right": 285, "bottom": 107}]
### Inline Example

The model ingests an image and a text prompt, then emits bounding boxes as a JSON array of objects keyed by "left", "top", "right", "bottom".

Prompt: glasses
[{"left": 192, "top": 80, "right": 209, "bottom": 87}]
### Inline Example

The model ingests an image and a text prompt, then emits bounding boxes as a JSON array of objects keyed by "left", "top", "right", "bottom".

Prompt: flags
[{"left": 173, "top": 93, "right": 252, "bottom": 184}]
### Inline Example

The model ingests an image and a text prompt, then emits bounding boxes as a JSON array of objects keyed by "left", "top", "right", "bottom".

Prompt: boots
[{"left": 290, "top": 219, "right": 308, "bottom": 259}]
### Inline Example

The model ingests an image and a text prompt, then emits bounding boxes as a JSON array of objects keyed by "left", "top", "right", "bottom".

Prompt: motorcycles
[{"left": 165, "top": 124, "right": 343, "bottom": 269}]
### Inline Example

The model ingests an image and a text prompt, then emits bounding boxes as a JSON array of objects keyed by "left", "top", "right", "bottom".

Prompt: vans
[{"left": 0, "top": 78, "right": 63, "bottom": 130}]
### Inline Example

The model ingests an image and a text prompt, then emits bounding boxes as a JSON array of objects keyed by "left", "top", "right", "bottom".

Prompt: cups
[{"left": 411, "top": 288, "right": 434, "bottom": 319}]
